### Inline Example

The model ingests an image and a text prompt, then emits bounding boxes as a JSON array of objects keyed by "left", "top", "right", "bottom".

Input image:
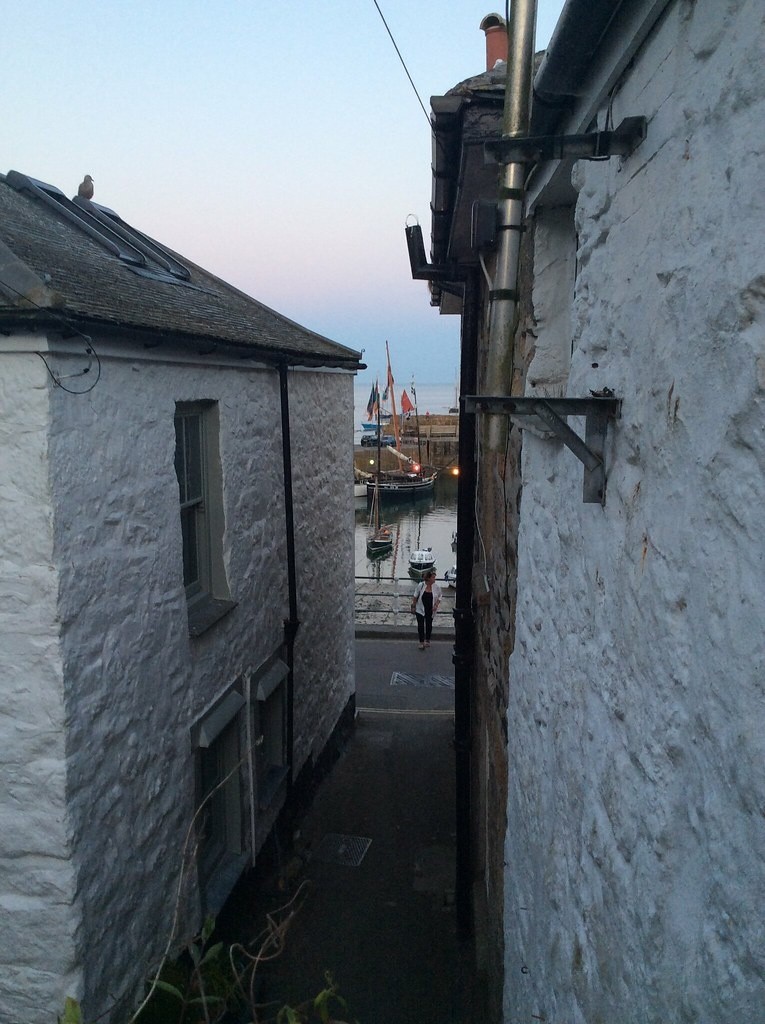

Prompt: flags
[
  {"left": 401, "top": 391, "right": 414, "bottom": 413},
  {"left": 367, "top": 388, "right": 378, "bottom": 420}
]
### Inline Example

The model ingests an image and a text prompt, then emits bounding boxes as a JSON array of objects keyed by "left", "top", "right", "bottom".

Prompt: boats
[
  {"left": 450, "top": 531, "right": 457, "bottom": 543},
  {"left": 361, "top": 418, "right": 390, "bottom": 430},
  {"left": 409, "top": 547, "right": 437, "bottom": 572},
  {"left": 448, "top": 387, "right": 459, "bottom": 413},
  {"left": 445, "top": 564, "right": 457, "bottom": 587}
]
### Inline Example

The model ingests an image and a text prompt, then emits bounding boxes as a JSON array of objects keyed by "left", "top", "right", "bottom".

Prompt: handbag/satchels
[{"left": 411, "top": 601, "right": 415, "bottom": 615}]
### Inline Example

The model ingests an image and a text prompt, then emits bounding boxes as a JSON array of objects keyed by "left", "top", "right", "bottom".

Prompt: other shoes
[
  {"left": 418, "top": 641, "right": 425, "bottom": 649},
  {"left": 424, "top": 640, "right": 430, "bottom": 648}
]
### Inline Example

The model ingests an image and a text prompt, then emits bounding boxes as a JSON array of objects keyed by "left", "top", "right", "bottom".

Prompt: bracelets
[{"left": 411, "top": 604, "right": 415, "bottom": 608}]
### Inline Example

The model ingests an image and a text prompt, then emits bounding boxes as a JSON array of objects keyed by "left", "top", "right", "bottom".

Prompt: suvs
[
  {"left": 381, "top": 435, "right": 395, "bottom": 446},
  {"left": 361, "top": 435, "right": 379, "bottom": 447}
]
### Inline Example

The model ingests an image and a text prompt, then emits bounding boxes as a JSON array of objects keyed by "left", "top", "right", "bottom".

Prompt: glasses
[{"left": 428, "top": 571, "right": 434, "bottom": 575}]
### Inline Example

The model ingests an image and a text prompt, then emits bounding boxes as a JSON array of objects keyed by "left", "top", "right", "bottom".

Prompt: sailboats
[
  {"left": 366, "top": 476, "right": 393, "bottom": 553},
  {"left": 366, "top": 340, "right": 441, "bottom": 497}
]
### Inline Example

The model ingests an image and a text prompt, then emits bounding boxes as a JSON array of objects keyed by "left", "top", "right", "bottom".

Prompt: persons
[
  {"left": 425, "top": 412, "right": 429, "bottom": 419},
  {"left": 411, "top": 572, "right": 442, "bottom": 650}
]
